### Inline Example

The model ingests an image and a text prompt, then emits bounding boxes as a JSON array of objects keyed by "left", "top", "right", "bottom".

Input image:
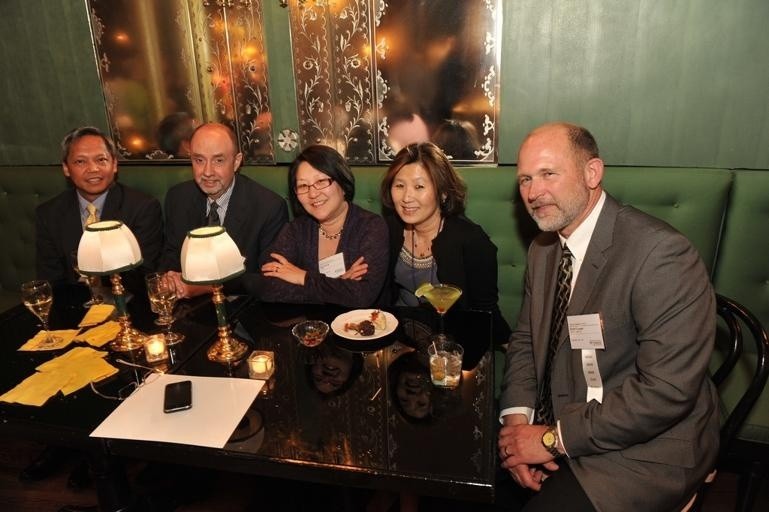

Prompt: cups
[{"left": 428, "top": 340, "right": 464, "bottom": 390}]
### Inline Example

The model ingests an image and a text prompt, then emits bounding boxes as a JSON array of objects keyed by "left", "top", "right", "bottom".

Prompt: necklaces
[
  {"left": 414, "top": 240, "right": 430, "bottom": 261},
  {"left": 410, "top": 216, "right": 444, "bottom": 296},
  {"left": 318, "top": 225, "right": 345, "bottom": 241}
]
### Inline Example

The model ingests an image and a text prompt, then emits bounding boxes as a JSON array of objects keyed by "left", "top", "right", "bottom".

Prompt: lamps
[
  {"left": 72, "top": 219, "right": 150, "bottom": 354},
  {"left": 178, "top": 223, "right": 252, "bottom": 366}
]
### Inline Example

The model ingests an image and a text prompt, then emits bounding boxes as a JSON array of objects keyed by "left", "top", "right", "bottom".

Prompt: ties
[
  {"left": 84, "top": 203, "right": 97, "bottom": 226},
  {"left": 208, "top": 202, "right": 220, "bottom": 226},
  {"left": 534, "top": 242, "right": 572, "bottom": 426}
]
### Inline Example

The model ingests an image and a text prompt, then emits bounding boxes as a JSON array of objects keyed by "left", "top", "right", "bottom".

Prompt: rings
[
  {"left": 502, "top": 444, "right": 509, "bottom": 455},
  {"left": 273, "top": 267, "right": 282, "bottom": 273}
]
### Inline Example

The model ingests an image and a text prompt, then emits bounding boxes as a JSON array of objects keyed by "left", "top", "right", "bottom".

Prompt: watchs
[{"left": 541, "top": 426, "right": 566, "bottom": 461}]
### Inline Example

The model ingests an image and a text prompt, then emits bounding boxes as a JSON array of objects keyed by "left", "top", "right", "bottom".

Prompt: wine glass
[
  {"left": 70, "top": 251, "right": 106, "bottom": 308},
  {"left": 18, "top": 276, "right": 64, "bottom": 350},
  {"left": 146, "top": 274, "right": 187, "bottom": 348},
  {"left": 420, "top": 282, "right": 462, "bottom": 354}
]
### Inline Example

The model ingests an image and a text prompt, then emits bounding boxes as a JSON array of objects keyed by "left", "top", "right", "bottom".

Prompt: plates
[{"left": 330, "top": 308, "right": 400, "bottom": 340}]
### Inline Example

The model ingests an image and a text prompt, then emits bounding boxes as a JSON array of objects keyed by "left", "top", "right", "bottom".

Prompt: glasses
[{"left": 293, "top": 178, "right": 334, "bottom": 194}]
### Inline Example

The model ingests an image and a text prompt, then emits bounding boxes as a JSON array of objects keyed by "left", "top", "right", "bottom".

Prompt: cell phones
[{"left": 163, "top": 381, "right": 192, "bottom": 412}]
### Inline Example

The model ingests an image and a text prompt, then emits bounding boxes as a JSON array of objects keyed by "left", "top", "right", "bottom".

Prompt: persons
[
  {"left": 377, "top": 142, "right": 513, "bottom": 343},
  {"left": 152, "top": 121, "right": 289, "bottom": 299},
  {"left": 256, "top": 146, "right": 390, "bottom": 308},
  {"left": 138, "top": 105, "right": 482, "bottom": 160},
  {"left": 499, "top": 123, "right": 721, "bottom": 510},
  {"left": 387, "top": 313, "right": 491, "bottom": 424},
  {"left": 264, "top": 303, "right": 366, "bottom": 397},
  {"left": 34, "top": 126, "right": 162, "bottom": 279}
]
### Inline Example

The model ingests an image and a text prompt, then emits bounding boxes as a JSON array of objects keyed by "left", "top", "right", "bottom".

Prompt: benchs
[
  {"left": 709, "top": 166, "right": 768, "bottom": 511},
  {"left": 1, "top": 160, "right": 734, "bottom": 418}
]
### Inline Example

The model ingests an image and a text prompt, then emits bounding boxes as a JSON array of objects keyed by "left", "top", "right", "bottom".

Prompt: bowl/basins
[{"left": 291, "top": 320, "right": 331, "bottom": 346}]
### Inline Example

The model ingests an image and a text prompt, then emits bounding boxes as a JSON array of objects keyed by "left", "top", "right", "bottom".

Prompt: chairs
[{"left": 682, "top": 282, "right": 768, "bottom": 512}]
